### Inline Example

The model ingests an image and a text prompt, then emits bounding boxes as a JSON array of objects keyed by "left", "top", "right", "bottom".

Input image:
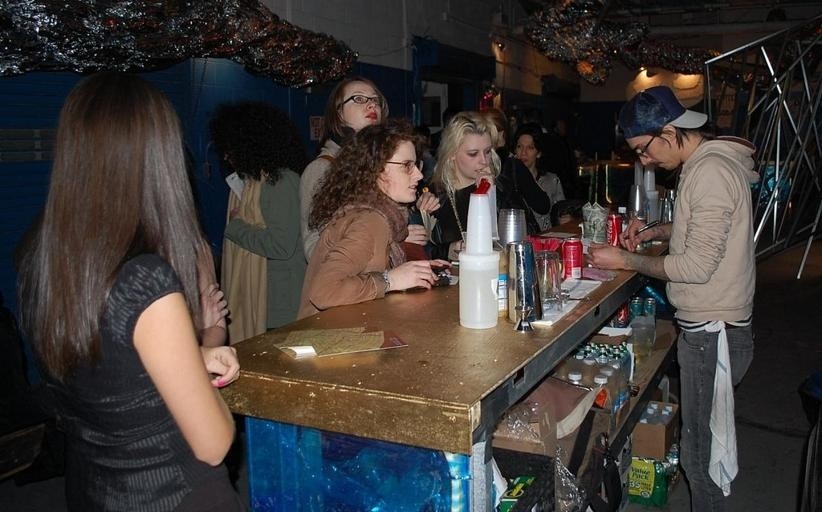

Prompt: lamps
[{"left": 629, "top": 67, "right": 707, "bottom": 110}]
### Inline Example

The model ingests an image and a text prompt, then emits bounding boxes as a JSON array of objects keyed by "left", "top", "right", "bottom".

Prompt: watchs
[{"left": 378, "top": 270, "right": 390, "bottom": 296}]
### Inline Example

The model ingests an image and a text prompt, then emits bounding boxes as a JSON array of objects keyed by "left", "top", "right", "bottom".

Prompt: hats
[{"left": 619, "top": 87, "right": 707, "bottom": 136}]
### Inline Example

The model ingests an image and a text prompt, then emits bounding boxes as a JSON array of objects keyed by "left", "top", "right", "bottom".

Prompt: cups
[
  {"left": 534, "top": 251, "right": 563, "bottom": 313},
  {"left": 498, "top": 209, "right": 527, "bottom": 248}
]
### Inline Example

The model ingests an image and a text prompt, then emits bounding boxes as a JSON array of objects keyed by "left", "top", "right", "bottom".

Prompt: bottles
[
  {"left": 551, "top": 342, "right": 635, "bottom": 431},
  {"left": 617, "top": 303, "right": 628, "bottom": 328},
  {"left": 507, "top": 241, "right": 542, "bottom": 322},
  {"left": 562, "top": 238, "right": 583, "bottom": 280},
  {"left": 638, "top": 399, "right": 674, "bottom": 427},
  {"left": 643, "top": 298, "right": 656, "bottom": 318},
  {"left": 606, "top": 215, "right": 623, "bottom": 246},
  {"left": 630, "top": 297, "right": 643, "bottom": 321},
  {"left": 659, "top": 188, "right": 677, "bottom": 224},
  {"left": 668, "top": 444, "right": 679, "bottom": 478},
  {"left": 618, "top": 207, "right": 629, "bottom": 232}
]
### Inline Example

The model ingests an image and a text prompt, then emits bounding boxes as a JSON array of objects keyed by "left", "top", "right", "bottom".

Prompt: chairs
[{"left": 2, "top": 301, "right": 73, "bottom": 512}]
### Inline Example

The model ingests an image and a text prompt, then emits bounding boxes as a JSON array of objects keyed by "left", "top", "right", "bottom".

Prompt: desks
[{"left": 208, "top": 209, "right": 681, "bottom": 510}]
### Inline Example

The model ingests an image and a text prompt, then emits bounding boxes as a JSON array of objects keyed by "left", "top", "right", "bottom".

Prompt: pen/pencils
[{"left": 624, "top": 220, "right": 659, "bottom": 240}]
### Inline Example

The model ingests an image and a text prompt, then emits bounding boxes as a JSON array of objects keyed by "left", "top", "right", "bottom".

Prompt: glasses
[
  {"left": 334, "top": 95, "right": 384, "bottom": 106},
  {"left": 630, "top": 137, "right": 654, "bottom": 161},
  {"left": 385, "top": 160, "right": 425, "bottom": 174}
]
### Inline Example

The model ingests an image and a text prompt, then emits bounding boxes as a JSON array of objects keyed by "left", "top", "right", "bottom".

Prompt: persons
[
  {"left": 211, "top": 103, "right": 306, "bottom": 345},
  {"left": 512, "top": 123, "right": 565, "bottom": 209},
  {"left": 587, "top": 88, "right": 761, "bottom": 512},
  {"left": 21, "top": 71, "right": 242, "bottom": 512},
  {"left": 299, "top": 79, "right": 429, "bottom": 266},
  {"left": 482, "top": 108, "right": 551, "bottom": 235},
  {"left": 295, "top": 123, "right": 440, "bottom": 320},
  {"left": 408, "top": 109, "right": 500, "bottom": 260}
]
